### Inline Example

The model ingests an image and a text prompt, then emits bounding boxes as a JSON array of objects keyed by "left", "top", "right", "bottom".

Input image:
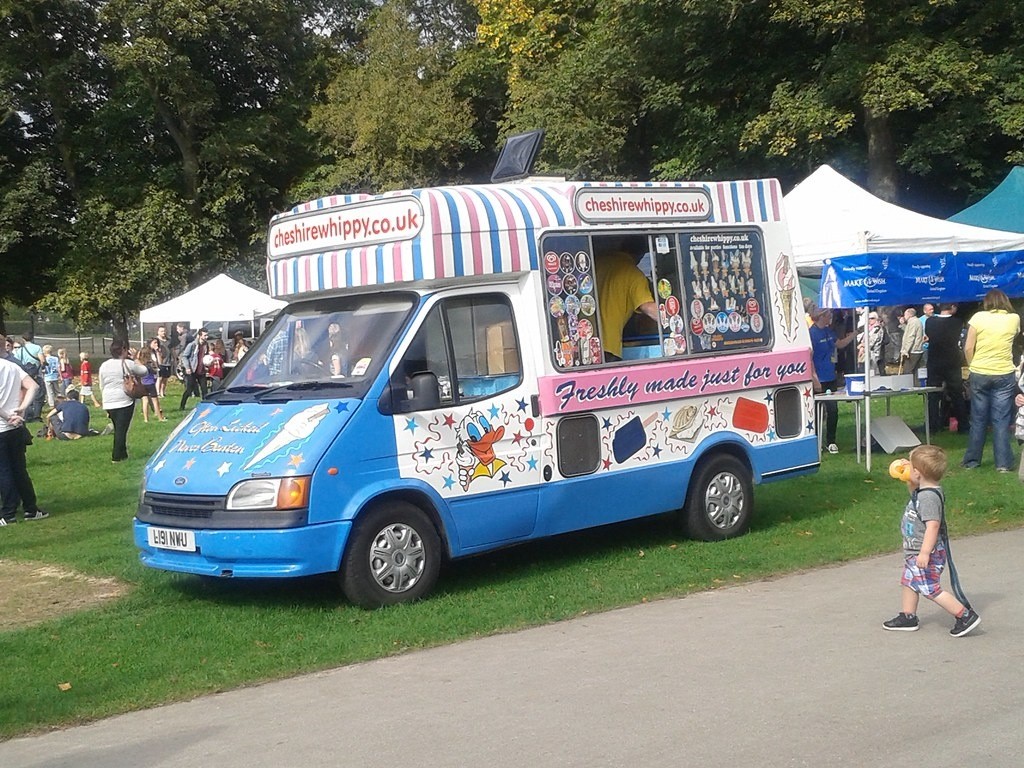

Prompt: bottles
[
  {"left": 960, "top": 327, "right": 968, "bottom": 339},
  {"left": 889, "top": 459, "right": 911, "bottom": 482},
  {"left": 37, "top": 425, "right": 55, "bottom": 440}
]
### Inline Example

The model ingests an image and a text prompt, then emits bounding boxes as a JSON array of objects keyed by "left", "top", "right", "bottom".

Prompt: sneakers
[
  {"left": 951, "top": 609, "right": 981, "bottom": 637},
  {"left": 23, "top": 508, "right": 49, "bottom": 521},
  {"left": 0, "top": 517, "right": 16, "bottom": 527},
  {"left": 882, "top": 612, "right": 919, "bottom": 630}
]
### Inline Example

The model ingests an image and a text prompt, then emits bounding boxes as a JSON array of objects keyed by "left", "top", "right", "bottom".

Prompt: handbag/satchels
[
  {"left": 25, "top": 362, "right": 39, "bottom": 377},
  {"left": 122, "top": 359, "right": 146, "bottom": 399}
]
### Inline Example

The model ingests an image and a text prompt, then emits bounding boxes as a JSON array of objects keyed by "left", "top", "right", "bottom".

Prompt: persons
[
  {"left": 809, "top": 308, "right": 864, "bottom": 456},
  {"left": 959, "top": 289, "right": 1021, "bottom": 473},
  {"left": 802, "top": 298, "right": 984, "bottom": 437},
  {"left": 0, "top": 304, "right": 100, "bottom": 520},
  {"left": 884, "top": 444, "right": 982, "bottom": 637},
  {"left": 592, "top": 235, "right": 659, "bottom": 364},
  {"left": 100, "top": 340, "right": 149, "bottom": 464},
  {"left": 134, "top": 323, "right": 319, "bottom": 423}
]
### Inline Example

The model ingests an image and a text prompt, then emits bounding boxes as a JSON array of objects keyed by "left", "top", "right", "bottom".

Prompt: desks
[{"left": 815, "top": 386, "right": 944, "bottom": 471}]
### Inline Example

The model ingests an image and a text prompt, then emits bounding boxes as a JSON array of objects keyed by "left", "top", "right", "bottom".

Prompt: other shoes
[
  {"left": 826, "top": 443, "right": 840, "bottom": 455},
  {"left": 998, "top": 467, "right": 1010, "bottom": 473}
]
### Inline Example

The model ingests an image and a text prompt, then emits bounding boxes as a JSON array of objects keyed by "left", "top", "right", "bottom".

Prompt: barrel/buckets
[{"left": 844, "top": 374, "right": 865, "bottom": 396}]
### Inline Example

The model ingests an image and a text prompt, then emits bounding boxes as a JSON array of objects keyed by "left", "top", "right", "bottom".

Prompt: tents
[
  {"left": 139, "top": 273, "right": 289, "bottom": 348},
  {"left": 740, "top": 163, "right": 1024, "bottom": 471},
  {"left": 945, "top": 166, "right": 1024, "bottom": 234}
]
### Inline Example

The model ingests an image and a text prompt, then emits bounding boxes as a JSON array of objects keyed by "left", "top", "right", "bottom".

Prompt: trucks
[{"left": 132, "top": 174, "right": 822, "bottom": 613}]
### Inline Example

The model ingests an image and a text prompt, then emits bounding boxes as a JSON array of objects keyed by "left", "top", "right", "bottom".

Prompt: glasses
[{"left": 870, "top": 317, "right": 877, "bottom": 320}]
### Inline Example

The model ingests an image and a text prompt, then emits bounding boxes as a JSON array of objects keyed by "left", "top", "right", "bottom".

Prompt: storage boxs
[
  {"left": 867, "top": 415, "right": 921, "bottom": 454},
  {"left": 484, "top": 323, "right": 520, "bottom": 373},
  {"left": 871, "top": 374, "right": 920, "bottom": 393},
  {"left": 844, "top": 374, "right": 867, "bottom": 395}
]
